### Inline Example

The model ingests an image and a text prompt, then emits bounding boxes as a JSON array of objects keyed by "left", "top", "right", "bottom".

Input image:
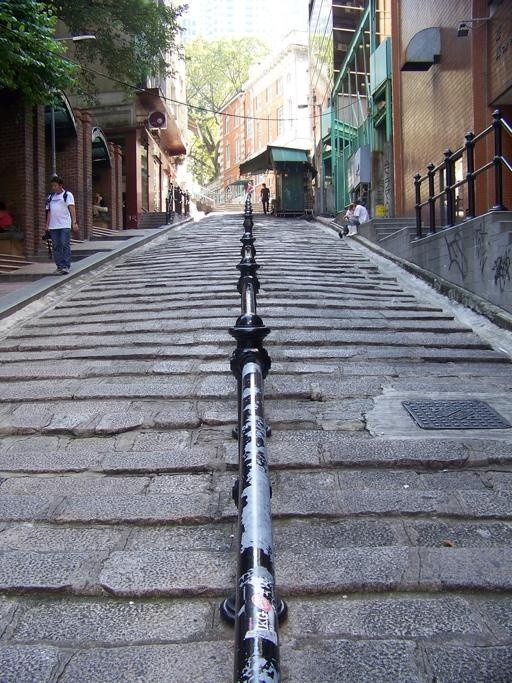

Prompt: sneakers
[{"left": 54, "top": 267, "right": 70, "bottom": 275}]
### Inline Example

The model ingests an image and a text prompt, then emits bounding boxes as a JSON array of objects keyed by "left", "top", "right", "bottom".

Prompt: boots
[{"left": 346, "top": 225, "right": 357, "bottom": 237}]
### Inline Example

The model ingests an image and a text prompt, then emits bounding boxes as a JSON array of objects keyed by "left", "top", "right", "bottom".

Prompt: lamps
[{"left": 455, "top": 3, "right": 499, "bottom": 37}]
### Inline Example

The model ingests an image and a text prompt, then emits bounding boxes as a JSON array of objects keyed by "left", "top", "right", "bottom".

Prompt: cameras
[{"left": 42, "top": 230, "right": 51, "bottom": 240}]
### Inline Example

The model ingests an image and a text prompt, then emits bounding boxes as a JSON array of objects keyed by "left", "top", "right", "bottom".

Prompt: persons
[
  {"left": 170, "top": 182, "right": 191, "bottom": 218},
  {"left": 260, "top": 183, "right": 270, "bottom": 214},
  {"left": 244, "top": 183, "right": 254, "bottom": 194},
  {"left": 0, "top": 204, "right": 13, "bottom": 233},
  {"left": 93, "top": 192, "right": 109, "bottom": 218},
  {"left": 44, "top": 176, "right": 79, "bottom": 275},
  {"left": 343, "top": 201, "right": 369, "bottom": 237}
]
